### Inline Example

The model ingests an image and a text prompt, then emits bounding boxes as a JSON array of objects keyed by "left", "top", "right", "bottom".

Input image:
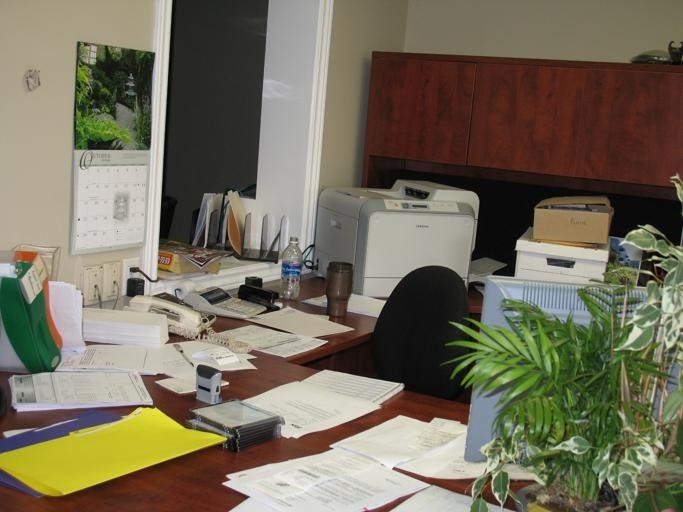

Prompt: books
[{"left": 302, "top": 369, "right": 406, "bottom": 404}]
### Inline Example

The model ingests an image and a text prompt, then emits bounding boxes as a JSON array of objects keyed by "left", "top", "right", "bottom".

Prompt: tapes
[{"left": 245, "top": 277, "right": 262, "bottom": 288}]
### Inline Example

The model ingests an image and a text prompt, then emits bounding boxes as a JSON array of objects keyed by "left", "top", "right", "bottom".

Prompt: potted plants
[{"left": 437, "top": 170, "right": 683, "bottom": 512}]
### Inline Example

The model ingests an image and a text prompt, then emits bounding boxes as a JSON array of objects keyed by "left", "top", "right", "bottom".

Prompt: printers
[{"left": 312, "top": 179, "right": 480, "bottom": 298}]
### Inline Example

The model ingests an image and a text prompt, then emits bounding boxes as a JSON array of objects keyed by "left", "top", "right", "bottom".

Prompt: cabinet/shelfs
[
  {"left": 200, "top": 271, "right": 483, "bottom": 405},
  {"left": 0, "top": 331, "right": 539, "bottom": 512},
  {"left": 576, "top": 60, "right": 683, "bottom": 202},
  {"left": 366, "top": 48, "right": 476, "bottom": 177},
  {"left": 468, "top": 55, "right": 576, "bottom": 188}
]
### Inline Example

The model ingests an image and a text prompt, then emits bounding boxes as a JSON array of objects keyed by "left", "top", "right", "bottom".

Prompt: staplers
[{"left": 238, "top": 285, "right": 279, "bottom": 311}]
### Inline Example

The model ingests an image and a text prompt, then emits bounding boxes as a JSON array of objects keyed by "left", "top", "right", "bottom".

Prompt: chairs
[{"left": 365, "top": 267, "right": 473, "bottom": 400}]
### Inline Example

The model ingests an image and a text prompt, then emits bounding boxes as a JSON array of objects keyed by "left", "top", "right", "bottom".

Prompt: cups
[{"left": 325, "top": 261, "right": 354, "bottom": 317}]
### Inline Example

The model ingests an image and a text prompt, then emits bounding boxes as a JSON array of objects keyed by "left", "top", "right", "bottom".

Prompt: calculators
[{"left": 184, "top": 286, "right": 267, "bottom": 319}]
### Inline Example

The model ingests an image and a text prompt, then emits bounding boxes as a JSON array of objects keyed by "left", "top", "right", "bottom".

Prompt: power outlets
[
  {"left": 101, "top": 260, "right": 121, "bottom": 302},
  {"left": 80, "top": 264, "right": 104, "bottom": 306},
  {"left": 120, "top": 257, "right": 141, "bottom": 296}
]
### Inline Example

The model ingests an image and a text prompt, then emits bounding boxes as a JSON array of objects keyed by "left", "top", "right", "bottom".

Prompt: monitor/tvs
[{"left": 464, "top": 275, "right": 683, "bottom": 512}]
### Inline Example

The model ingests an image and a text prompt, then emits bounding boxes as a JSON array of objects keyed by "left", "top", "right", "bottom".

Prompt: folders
[{"left": 0, "top": 413, "right": 123, "bottom": 496}]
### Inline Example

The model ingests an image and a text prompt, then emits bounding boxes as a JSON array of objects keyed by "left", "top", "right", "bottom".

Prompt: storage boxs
[{"left": 513, "top": 197, "right": 614, "bottom": 285}]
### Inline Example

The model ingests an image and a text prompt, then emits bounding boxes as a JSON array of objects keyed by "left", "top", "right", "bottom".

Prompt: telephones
[{"left": 129, "top": 292, "right": 206, "bottom": 336}]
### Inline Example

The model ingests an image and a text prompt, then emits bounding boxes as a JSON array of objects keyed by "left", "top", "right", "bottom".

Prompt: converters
[{"left": 127, "top": 278, "right": 144, "bottom": 298}]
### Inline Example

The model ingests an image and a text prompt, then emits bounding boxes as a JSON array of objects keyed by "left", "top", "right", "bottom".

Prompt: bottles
[{"left": 277, "top": 238, "right": 301, "bottom": 298}]
